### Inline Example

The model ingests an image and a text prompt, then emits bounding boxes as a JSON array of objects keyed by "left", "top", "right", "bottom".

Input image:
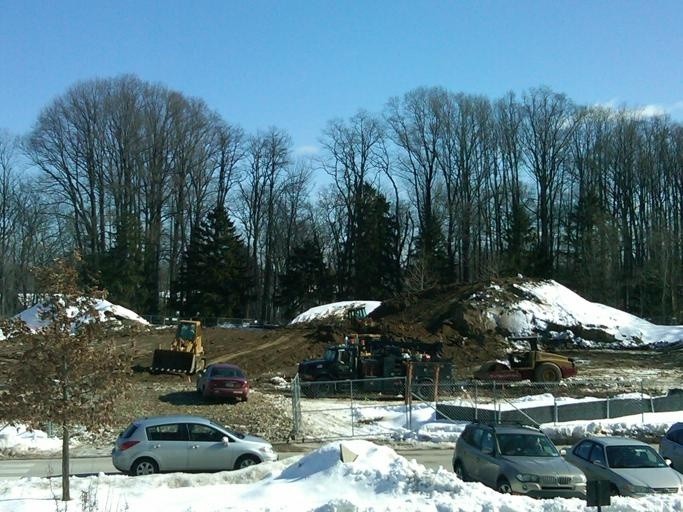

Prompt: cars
[
  {"left": 194, "top": 362, "right": 250, "bottom": 402},
  {"left": 657, "top": 420, "right": 683, "bottom": 474},
  {"left": 110, "top": 414, "right": 281, "bottom": 476},
  {"left": 160, "top": 317, "right": 282, "bottom": 330},
  {"left": 558, "top": 435, "right": 683, "bottom": 497}
]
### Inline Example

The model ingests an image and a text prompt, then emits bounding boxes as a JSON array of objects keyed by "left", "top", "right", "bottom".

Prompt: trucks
[{"left": 293, "top": 340, "right": 455, "bottom": 400}]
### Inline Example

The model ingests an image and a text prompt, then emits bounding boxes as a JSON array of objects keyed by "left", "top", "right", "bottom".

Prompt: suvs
[{"left": 447, "top": 418, "right": 587, "bottom": 501}]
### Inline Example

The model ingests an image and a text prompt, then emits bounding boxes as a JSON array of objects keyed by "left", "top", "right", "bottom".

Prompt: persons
[
  {"left": 422, "top": 352, "right": 431, "bottom": 362},
  {"left": 414, "top": 351, "right": 422, "bottom": 362},
  {"left": 185, "top": 324, "right": 194, "bottom": 336},
  {"left": 399, "top": 348, "right": 411, "bottom": 359}
]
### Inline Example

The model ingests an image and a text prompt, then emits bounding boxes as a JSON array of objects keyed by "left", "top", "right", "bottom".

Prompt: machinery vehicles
[
  {"left": 473, "top": 335, "right": 578, "bottom": 391},
  {"left": 345, "top": 305, "right": 385, "bottom": 343},
  {"left": 150, "top": 317, "right": 208, "bottom": 376}
]
